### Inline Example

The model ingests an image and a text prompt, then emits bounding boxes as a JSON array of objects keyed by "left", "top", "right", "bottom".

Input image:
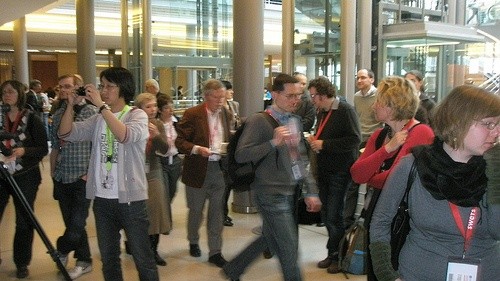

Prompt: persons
[
  {"left": 369, "top": 85, "right": 500, "bottom": 281},
  {"left": 344, "top": 68, "right": 383, "bottom": 229},
  {"left": 349, "top": 76, "right": 434, "bottom": 281},
  {"left": 57, "top": 66, "right": 160, "bottom": 281},
  {"left": 0, "top": 79, "right": 49, "bottom": 278},
  {"left": 222, "top": 80, "right": 239, "bottom": 227},
  {"left": 221, "top": 73, "right": 308, "bottom": 281},
  {"left": 294, "top": 72, "right": 316, "bottom": 131},
  {"left": 308, "top": 78, "right": 361, "bottom": 273},
  {"left": 158, "top": 94, "right": 181, "bottom": 199},
  {"left": 178, "top": 85, "right": 183, "bottom": 100},
  {"left": 175, "top": 79, "right": 231, "bottom": 267},
  {"left": 49, "top": 74, "right": 98, "bottom": 278},
  {"left": 145, "top": 79, "right": 173, "bottom": 119},
  {"left": 125, "top": 94, "right": 172, "bottom": 265},
  {"left": 27, "top": 80, "right": 60, "bottom": 116},
  {"left": 406, "top": 70, "right": 436, "bottom": 126}
]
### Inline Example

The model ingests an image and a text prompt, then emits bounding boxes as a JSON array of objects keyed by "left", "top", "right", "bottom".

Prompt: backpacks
[{"left": 218, "top": 111, "right": 281, "bottom": 193}]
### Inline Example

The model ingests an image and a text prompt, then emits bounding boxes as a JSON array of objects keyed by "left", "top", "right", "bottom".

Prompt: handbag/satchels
[
  {"left": 368, "top": 207, "right": 411, "bottom": 281},
  {"left": 296, "top": 197, "right": 326, "bottom": 227},
  {"left": 339, "top": 221, "right": 369, "bottom": 276}
]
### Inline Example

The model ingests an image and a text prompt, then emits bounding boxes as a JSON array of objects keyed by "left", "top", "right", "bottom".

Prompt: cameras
[{"left": 77, "top": 86, "right": 87, "bottom": 96}]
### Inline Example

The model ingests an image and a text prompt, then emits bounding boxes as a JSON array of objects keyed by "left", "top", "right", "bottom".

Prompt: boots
[{"left": 150, "top": 234, "right": 167, "bottom": 267}]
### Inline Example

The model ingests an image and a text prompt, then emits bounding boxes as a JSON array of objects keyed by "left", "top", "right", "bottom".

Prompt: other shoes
[
  {"left": 15, "top": 264, "right": 29, "bottom": 278},
  {"left": 221, "top": 267, "right": 240, "bottom": 281},
  {"left": 208, "top": 253, "right": 229, "bottom": 268},
  {"left": 318, "top": 257, "right": 340, "bottom": 274},
  {"left": 68, "top": 261, "right": 92, "bottom": 280},
  {"left": 56, "top": 249, "right": 68, "bottom": 268},
  {"left": 224, "top": 216, "right": 233, "bottom": 226},
  {"left": 189, "top": 243, "right": 201, "bottom": 258}
]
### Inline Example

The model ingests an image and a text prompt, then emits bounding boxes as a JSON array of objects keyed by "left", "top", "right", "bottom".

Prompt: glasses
[
  {"left": 473, "top": 119, "right": 500, "bottom": 130},
  {"left": 310, "top": 93, "right": 319, "bottom": 98},
  {"left": 2, "top": 91, "right": 18, "bottom": 95},
  {"left": 98, "top": 84, "right": 120, "bottom": 90},
  {"left": 56, "top": 84, "right": 74, "bottom": 90},
  {"left": 357, "top": 76, "right": 371, "bottom": 80}
]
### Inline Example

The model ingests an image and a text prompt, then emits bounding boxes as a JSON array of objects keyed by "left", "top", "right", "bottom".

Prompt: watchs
[{"left": 98, "top": 104, "right": 111, "bottom": 114}]
